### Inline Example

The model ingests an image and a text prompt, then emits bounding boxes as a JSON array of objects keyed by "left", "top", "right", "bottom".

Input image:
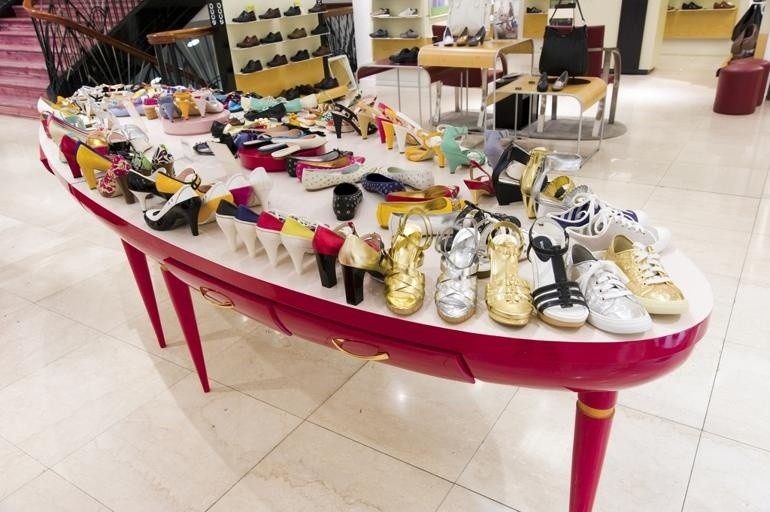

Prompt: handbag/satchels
[
  {"left": 490, "top": 15, "right": 590, "bottom": 76},
  {"left": 731, "top": 5, "right": 762, "bottom": 58}
]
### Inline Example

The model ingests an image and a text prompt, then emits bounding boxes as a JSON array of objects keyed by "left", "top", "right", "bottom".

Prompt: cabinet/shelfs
[
  {"left": 372, "top": 1, "right": 427, "bottom": 70},
  {"left": 664, "top": 1, "right": 738, "bottom": 39},
  {"left": 206, "top": 0, "right": 348, "bottom": 104}
]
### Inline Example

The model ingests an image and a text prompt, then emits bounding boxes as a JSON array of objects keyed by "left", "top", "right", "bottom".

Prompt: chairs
[
  {"left": 434, "top": 26, "right": 507, "bottom": 122},
  {"left": 546, "top": 25, "right": 622, "bottom": 127}
]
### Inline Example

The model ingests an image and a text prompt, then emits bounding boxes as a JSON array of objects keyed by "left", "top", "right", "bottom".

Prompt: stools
[
  {"left": 356, "top": 51, "right": 455, "bottom": 133},
  {"left": 730, "top": 59, "right": 770, "bottom": 106},
  {"left": 713, "top": 63, "right": 764, "bottom": 115},
  {"left": 481, "top": 71, "right": 608, "bottom": 169},
  {"left": 417, "top": 38, "right": 535, "bottom": 149}
]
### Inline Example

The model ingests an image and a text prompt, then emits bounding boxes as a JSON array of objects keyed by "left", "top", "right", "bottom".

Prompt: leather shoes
[
  {"left": 537, "top": 70, "right": 569, "bottom": 91},
  {"left": 527, "top": 6, "right": 541, "bottom": 13},
  {"left": 232, "top": 4, "right": 339, "bottom": 101},
  {"left": 667, "top": 0, "right": 736, "bottom": 10}
]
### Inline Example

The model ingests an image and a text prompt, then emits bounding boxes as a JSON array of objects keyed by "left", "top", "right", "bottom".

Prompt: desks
[{"left": 37, "top": 86, "right": 715, "bottom": 512}]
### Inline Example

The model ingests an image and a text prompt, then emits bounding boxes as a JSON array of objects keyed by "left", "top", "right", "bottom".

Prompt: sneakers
[{"left": 369, "top": 8, "right": 419, "bottom": 63}]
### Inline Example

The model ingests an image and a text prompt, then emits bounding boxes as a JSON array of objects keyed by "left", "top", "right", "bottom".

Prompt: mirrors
[{"left": 328, "top": 54, "right": 357, "bottom": 91}]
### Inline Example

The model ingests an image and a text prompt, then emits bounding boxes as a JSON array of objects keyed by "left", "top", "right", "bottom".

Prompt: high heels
[{"left": 443, "top": 26, "right": 486, "bottom": 46}]
